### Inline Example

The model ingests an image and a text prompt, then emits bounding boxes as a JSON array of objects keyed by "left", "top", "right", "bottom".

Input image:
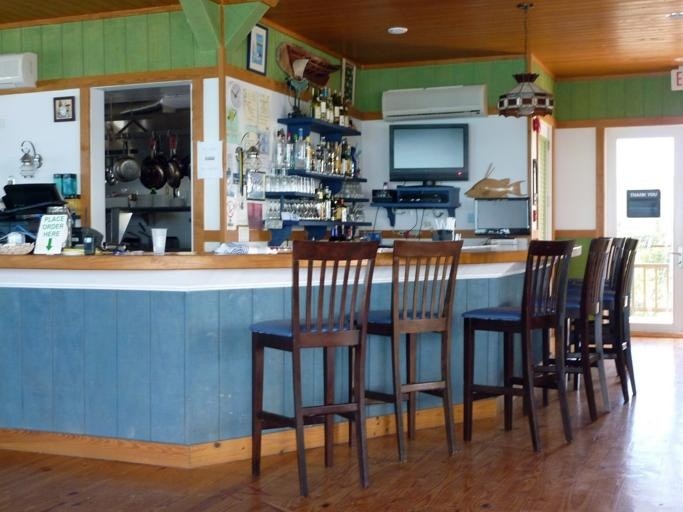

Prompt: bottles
[
  {"left": 330, "top": 223, "right": 346, "bottom": 241},
  {"left": 276, "top": 86, "right": 357, "bottom": 222},
  {"left": 62, "top": 174, "right": 77, "bottom": 198},
  {"left": 53, "top": 174, "right": 63, "bottom": 198}
]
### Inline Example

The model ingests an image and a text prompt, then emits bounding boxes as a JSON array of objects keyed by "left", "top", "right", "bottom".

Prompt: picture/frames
[
  {"left": 340, "top": 58, "right": 357, "bottom": 105},
  {"left": 246, "top": 24, "right": 268, "bottom": 75},
  {"left": 52, "top": 96, "right": 75, "bottom": 122},
  {"left": 246, "top": 169, "right": 266, "bottom": 201}
]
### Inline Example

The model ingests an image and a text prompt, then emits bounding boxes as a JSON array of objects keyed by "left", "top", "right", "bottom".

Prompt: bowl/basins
[{"left": 0, "top": 243, "right": 34, "bottom": 255}]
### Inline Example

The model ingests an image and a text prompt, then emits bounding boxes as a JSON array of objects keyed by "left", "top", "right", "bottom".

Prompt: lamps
[
  {"left": 497, "top": 4, "right": 556, "bottom": 119},
  {"left": 17, "top": 138, "right": 41, "bottom": 179}
]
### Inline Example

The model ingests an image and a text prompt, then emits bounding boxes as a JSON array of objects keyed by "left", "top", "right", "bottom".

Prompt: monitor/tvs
[{"left": 388, "top": 123, "right": 469, "bottom": 188}]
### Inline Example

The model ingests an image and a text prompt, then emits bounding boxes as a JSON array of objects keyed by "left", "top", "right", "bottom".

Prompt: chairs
[
  {"left": 536, "top": 236, "right": 612, "bottom": 419},
  {"left": 248, "top": 239, "right": 380, "bottom": 497},
  {"left": 463, "top": 239, "right": 578, "bottom": 452},
  {"left": 339, "top": 240, "right": 464, "bottom": 461},
  {"left": 567, "top": 236, "right": 627, "bottom": 390},
  {"left": 537, "top": 237, "right": 639, "bottom": 405}
]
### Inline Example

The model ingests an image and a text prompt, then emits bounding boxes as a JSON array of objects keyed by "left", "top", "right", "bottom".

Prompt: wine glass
[
  {"left": 226, "top": 201, "right": 234, "bottom": 227},
  {"left": 223, "top": 170, "right": 233, "bottom": 195}
]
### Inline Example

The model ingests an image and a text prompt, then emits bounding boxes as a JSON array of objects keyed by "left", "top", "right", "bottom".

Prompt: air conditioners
[
  {"left": 1, "top": 53, "right": 38, "bottom": 89},
  {"left": 380, "top": 84, "right": 489, "bottom": 121}
]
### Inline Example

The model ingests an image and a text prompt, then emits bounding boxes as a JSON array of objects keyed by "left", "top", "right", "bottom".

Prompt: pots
[{"left": 105, "top": 128, "right": 191, "bottom": 190}]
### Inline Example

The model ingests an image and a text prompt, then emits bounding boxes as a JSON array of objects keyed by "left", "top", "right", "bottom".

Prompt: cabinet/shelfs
[{"left": 271, "top": 117, "right": 372, "bottom": 248}]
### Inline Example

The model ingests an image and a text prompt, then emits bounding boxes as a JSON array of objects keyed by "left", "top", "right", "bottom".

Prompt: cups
[
  {"left": 82, "top": 229, "right": 95, "bottom": 255},
  {"left": 363, "top": 232, "right": 381, "bottom": 244},
  {"left": 431, "top": 229, "right": 452, "bottom": 240},
  {"left": 151, "top": 228, "right": 167, "bottom": 255},
  {"left": 264, "top": 175, "right": 318, "bottom": 220},
  {"left": 7, "top": 230, "right": 25, "bottom": 243}
]
